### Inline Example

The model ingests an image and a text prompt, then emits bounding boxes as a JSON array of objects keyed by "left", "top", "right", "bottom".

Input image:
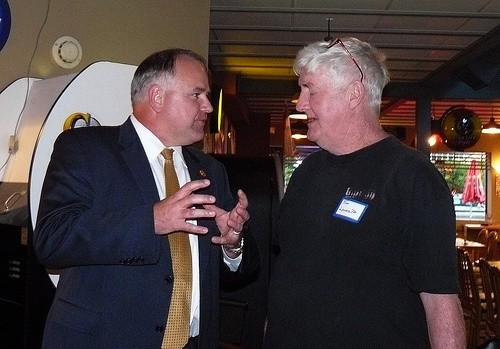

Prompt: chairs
[{"left": 456, "top": 228, "right": 500, "bottom": 349}]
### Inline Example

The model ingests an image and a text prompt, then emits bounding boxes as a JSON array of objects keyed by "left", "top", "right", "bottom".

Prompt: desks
[
  {"left": 456, "top": 238, "right": 486, "bottom": 248},
  {"left": 464, "top": 224, "right": 500, "bottom": 240}
]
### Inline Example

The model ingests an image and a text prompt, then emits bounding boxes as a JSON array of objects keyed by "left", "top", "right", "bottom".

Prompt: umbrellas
[{"left": 459, "top": 160, "right": 486, "bottom": 218}]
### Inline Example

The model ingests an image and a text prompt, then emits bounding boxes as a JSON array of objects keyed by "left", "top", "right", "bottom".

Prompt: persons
[
  {"left": 255, "top": 34, "right": 467, "bottom": 349},
  {"left": 32, "top": 48, "right": 261, "bottom": 349}
]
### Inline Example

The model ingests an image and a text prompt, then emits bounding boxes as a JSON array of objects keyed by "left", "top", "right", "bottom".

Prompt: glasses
[{"left": 325, "top": 35, "right": 364, "bottom": 84}]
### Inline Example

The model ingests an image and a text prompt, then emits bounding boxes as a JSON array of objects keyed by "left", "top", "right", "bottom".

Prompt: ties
[{"left": 158, "top": 147, "right": 192, "bottom": 348}]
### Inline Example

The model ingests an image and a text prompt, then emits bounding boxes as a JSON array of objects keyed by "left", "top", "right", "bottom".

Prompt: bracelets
[{"left": 223, "top": 236, "right": 245, "bottom": 253}]
